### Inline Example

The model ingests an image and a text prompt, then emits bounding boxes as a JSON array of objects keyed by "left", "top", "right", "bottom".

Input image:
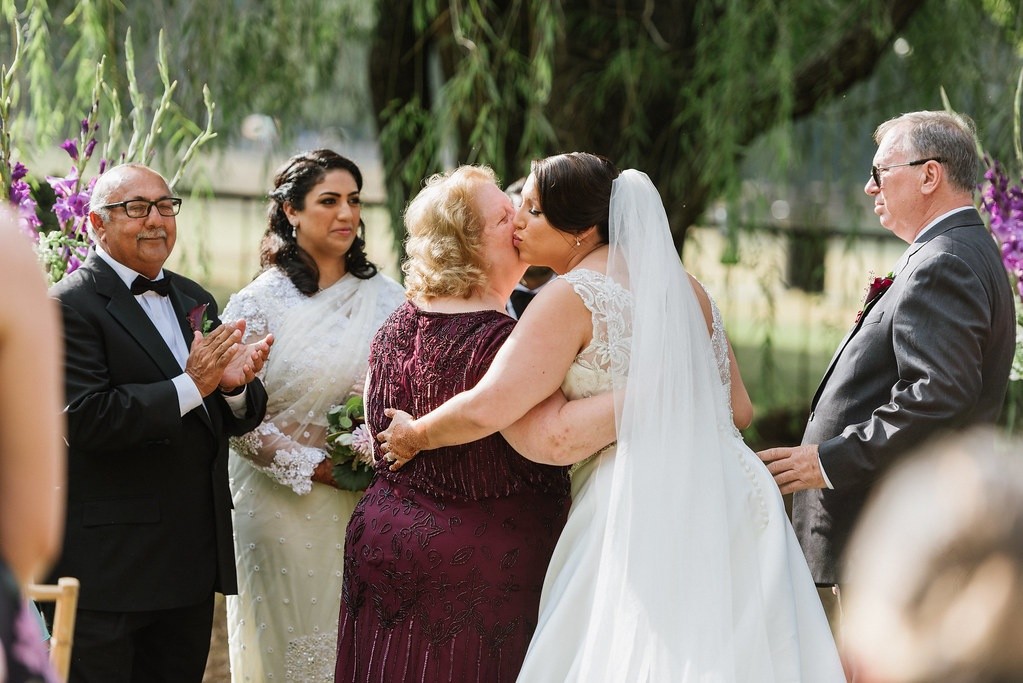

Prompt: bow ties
[{"left": 130, "top": 275, "right": 173, "bottom": 298}]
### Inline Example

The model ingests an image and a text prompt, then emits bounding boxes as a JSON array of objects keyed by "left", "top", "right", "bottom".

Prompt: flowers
[
  {"left": 0, "top": 17, "right": 219, "bottom": 286},
  {"left": 939, "top": 70, "right": 1023, "bottom": 309},
  {"left": 185, "top": 302, "right": 211, "bottom": 333},
  {"left": 327, "top": 392, "right": 380, "bottom": 490},
  {"left": 853, "top": 270, "right": 897, "bottom": 321}
]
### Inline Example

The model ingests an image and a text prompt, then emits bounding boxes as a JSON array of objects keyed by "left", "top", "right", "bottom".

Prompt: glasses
[
  {"left": 870, "top": 156, "right": 949, "bottom": 188},
  {"left": 98, "top": 198, "right": 182, "bottom": 218}
]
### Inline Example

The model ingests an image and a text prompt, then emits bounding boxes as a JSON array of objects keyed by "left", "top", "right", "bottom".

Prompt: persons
[
  {"left": 378, "top": 153, "right": 846, "bottom": 682},
  {"left": 218, "top": 149, "right": 407, "bottom": 682},
  {"left": 333, "top": 165, "right": 625, "bottom": 682},
  {"left": 0, "top": 163, "right": 275, "bottom": 682},
  {"left": 750, "top": 110, "right": 1015, "bottom": 586}
]
